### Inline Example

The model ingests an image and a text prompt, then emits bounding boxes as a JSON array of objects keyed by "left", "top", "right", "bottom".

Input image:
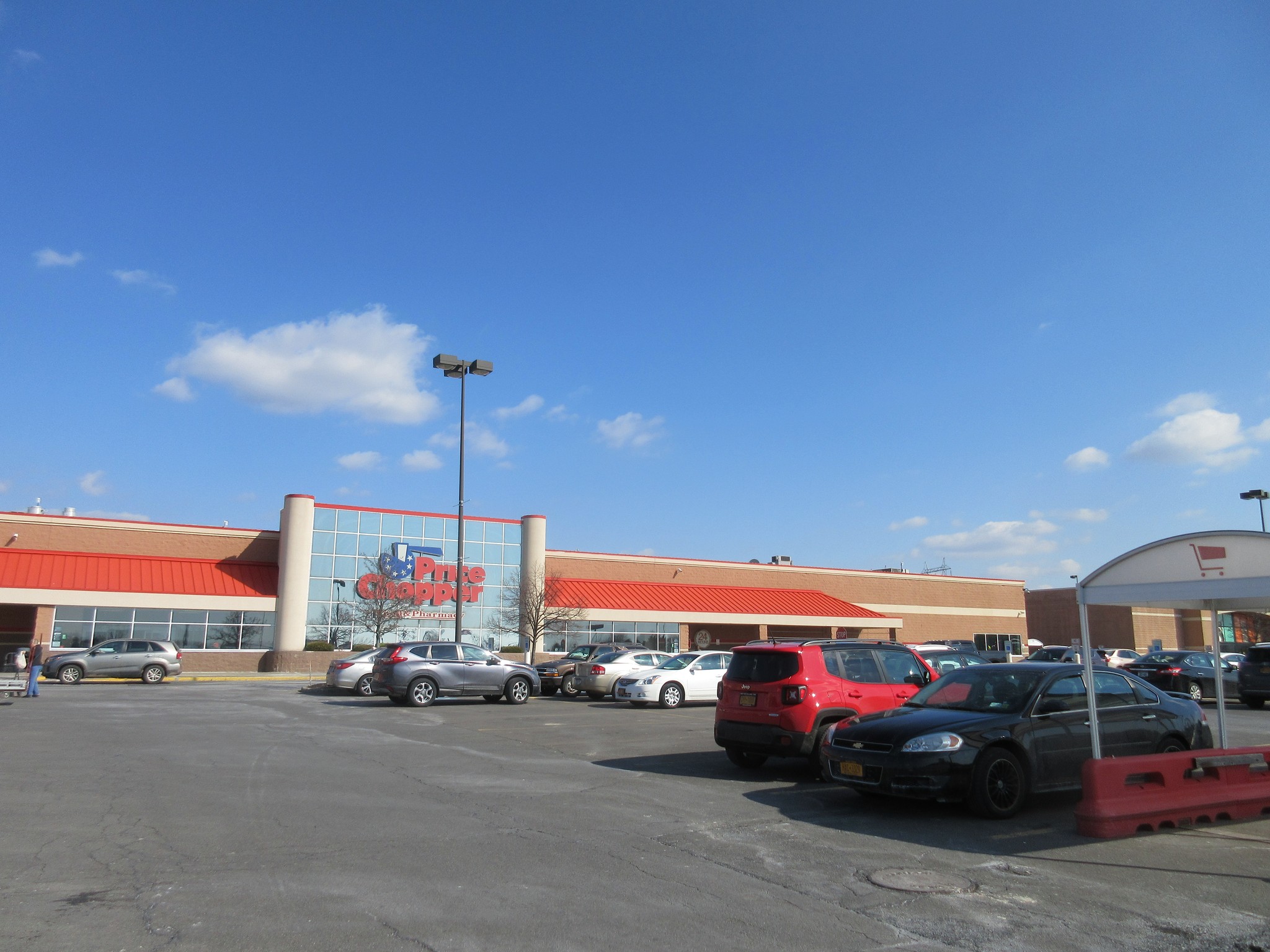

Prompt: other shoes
[
  {"left": 23, "top": 695, "right": 32, "bottom": 698},
  {"left": 32, "top": 694, "right": 40, "bottom": 697}
]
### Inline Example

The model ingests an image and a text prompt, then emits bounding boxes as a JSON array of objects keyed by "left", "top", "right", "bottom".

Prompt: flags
[{"left": 461, "top": 630, "right": 472, "bottom": 635}]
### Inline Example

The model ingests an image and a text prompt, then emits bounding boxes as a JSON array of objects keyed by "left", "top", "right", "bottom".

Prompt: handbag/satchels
[{"left": 17, "top": 651, "right": 28, "bottom": 669}]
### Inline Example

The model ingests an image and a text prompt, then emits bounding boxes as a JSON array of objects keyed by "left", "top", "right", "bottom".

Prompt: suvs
[
  {"left": 712, "top": 638, "right": 971, "bottom": 785},
  {"left": 369, "top": 640, "right": 541, "bottom": 707},
  {"left": 40, "top": 638, "right": 183, "bottom": 684},
  {"left": 1237, "top": 642, "right": 1270, "bottom": 708},
  {"left": 532, "top": 642, "right": 651, "bottom": 697},
  {"left": 1017, "top": 646, "right": 1108, "bottom": 689}
]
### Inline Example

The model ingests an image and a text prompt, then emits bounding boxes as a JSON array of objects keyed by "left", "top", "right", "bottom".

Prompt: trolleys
[{"left": 0, "top": 652, "right": 31, "bottom": 698}]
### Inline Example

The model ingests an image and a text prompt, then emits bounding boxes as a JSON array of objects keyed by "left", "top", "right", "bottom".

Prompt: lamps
[
  {"left": 677, "top": 568, "right": 682, "bottom": 573},
  {"left": 1018, "top": 611, "right": 1025, "bottom": 614},
  {"left": 1022, "top": 588, "right": 1030, "bottom": 593},
  {"left": 1070, "top": 575, "right": 1078, "bottom": 583},
  {"left": 12, "top": 534, "right": 18, "bottom": 541}
]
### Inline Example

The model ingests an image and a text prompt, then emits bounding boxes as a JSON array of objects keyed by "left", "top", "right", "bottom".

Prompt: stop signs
[{"left": 836, "top": 627, "right": 847, "bottom": 639}]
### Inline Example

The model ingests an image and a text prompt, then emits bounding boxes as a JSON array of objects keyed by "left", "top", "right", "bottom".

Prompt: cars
[
  {"left": 1122, "top": 650, "right": 1241, "bottom": 704},
  {"left": 615, "top": 650, "right": 733, "bottom": 709},
  {"left": 1094, "top": 648, "right": 1142, "bottom": 672},
  {"left": 571, "top": 649, "right": 688, "bottom": 700},
  {"left": 818, "top": 661, "right": 1215, "bottom": 821},
  {"left": 1210, "top": 652, "right": 1246, "bottom": 667},
  {"left": 325, "top": 647, "right": 386, "bottom": 697},
  {"left": 901, "top": 639, "right": 1007, "bottom": 677}
]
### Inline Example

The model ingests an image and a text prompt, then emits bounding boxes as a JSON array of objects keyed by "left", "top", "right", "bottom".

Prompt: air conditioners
[{"left": 772, "top": 556, "right": 791, "bottom": 566}]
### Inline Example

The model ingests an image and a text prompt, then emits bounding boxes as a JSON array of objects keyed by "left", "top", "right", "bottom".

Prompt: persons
[{"left": 22, "top": 638, "right": 44, "bottom": 698}]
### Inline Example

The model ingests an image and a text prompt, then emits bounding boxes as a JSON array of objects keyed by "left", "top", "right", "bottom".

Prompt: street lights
[
  {"left": 333, "top": 579, "right": 346, "bottom": 626},
  {"left": 1239, "top": 489, "right": 1270, "bottom": 533},
  {"left": 432, "top": 354, "right": 495, "bottom": 642},
  {"left": 1070, "top": 575, "right": 1078, "bottom": 583}
]
